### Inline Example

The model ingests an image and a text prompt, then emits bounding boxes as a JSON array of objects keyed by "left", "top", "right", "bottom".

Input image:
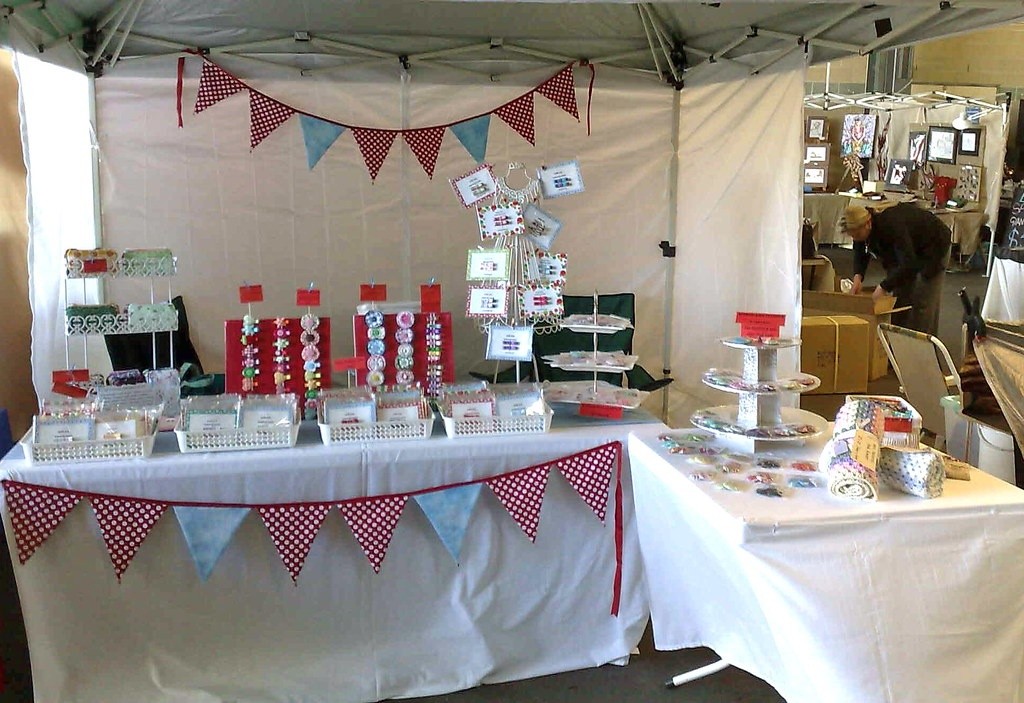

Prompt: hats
[{"left": 840, "top": 204, "right": 871, "bottom": 232}]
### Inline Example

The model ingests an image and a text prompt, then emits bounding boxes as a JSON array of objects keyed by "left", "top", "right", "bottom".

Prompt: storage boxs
[{"left": 799, "top": 288, "right": 914, "bottom": 394}]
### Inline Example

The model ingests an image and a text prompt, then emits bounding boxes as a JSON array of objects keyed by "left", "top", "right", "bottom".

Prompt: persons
[{"left": 836, "top": 202, "right": 952, "bottom": 375}]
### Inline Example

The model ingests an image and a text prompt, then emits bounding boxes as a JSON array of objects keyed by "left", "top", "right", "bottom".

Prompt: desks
[
  {"left": 0, "top": 381, "right": 1024, "bottom": 701},
  {"left": 803, "top": 192, "right": 986, "bottom": 274}
]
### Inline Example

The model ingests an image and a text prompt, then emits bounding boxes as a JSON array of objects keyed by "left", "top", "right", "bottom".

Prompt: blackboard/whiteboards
[{"left": 1001, "top": 186, "right": 1024, "bottom": 248}]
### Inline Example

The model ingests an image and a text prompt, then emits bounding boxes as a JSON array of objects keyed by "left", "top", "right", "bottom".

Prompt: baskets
[
  {"left": 174, "top": 414, "right": 302, "bottom": 453},
  {"left": 19, "top": 418, "right": 161, "bottom": 464},
  {"left": 440, "top": 399, "right": 555, "bottom": 437},
  {"left": 317, "top": 404, "right": 436, "bottom": 445}
]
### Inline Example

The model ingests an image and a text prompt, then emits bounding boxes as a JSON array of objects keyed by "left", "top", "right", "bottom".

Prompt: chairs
[
  {"left": 975, "top": 335, "right": 1023, "bottom": 456},
  {"left": 877, "top": 323, "right": 963, "bottom": 455},
  {"left": 105, "top": 291, "right": 225, "bottom": 400},
  {"left": 533, "top": 293, "right": 671, "bottom": 428}
]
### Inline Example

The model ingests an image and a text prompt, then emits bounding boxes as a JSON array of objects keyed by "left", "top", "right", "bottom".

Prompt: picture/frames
[
  {"left": 924, "top": 127, "right": 958, "bottom": 165},
  {"left": 804, "top": 115, "right": 832, "bottom": 188},
  {"left": 960, "top": 128, "right": 980, "bottom": 156}
]
